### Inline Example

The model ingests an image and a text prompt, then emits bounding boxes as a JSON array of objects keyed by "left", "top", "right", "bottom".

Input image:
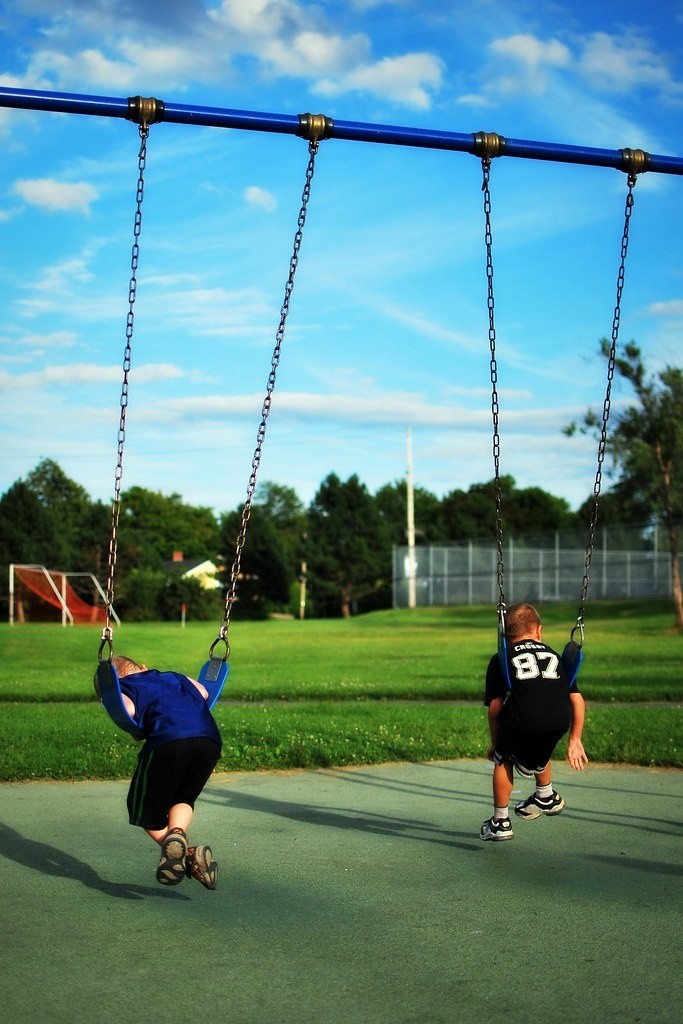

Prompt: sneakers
[
  {"left": 480, "top": 816, "right": 514, "bottom": 841},
  {"left": 514, "top": 790, "right": 565, "bottom": 820}
]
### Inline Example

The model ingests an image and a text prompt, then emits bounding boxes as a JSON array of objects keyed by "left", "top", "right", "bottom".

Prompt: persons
[
  {"left": 480, "top": 603, "right": 589, "bottom": 842},
  {"left": 108, "top": 655, "right": 222, "bottom": 891}
]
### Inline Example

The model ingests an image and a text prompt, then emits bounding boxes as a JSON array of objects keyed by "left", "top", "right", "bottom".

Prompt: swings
[
  {"left": 483, "top": 153, "right": 639, "bottom": 692},
  {"left": 93, "top": 116, "right": 317, "bottom": 737}
]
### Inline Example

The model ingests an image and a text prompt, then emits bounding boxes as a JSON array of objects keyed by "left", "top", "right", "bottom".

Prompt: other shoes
[
  {"left": 156, "top": 828, "right": 188, "bottom": 886},
  {"left": 185, "top": 846, "right": 218, "bottom": 890}
]
